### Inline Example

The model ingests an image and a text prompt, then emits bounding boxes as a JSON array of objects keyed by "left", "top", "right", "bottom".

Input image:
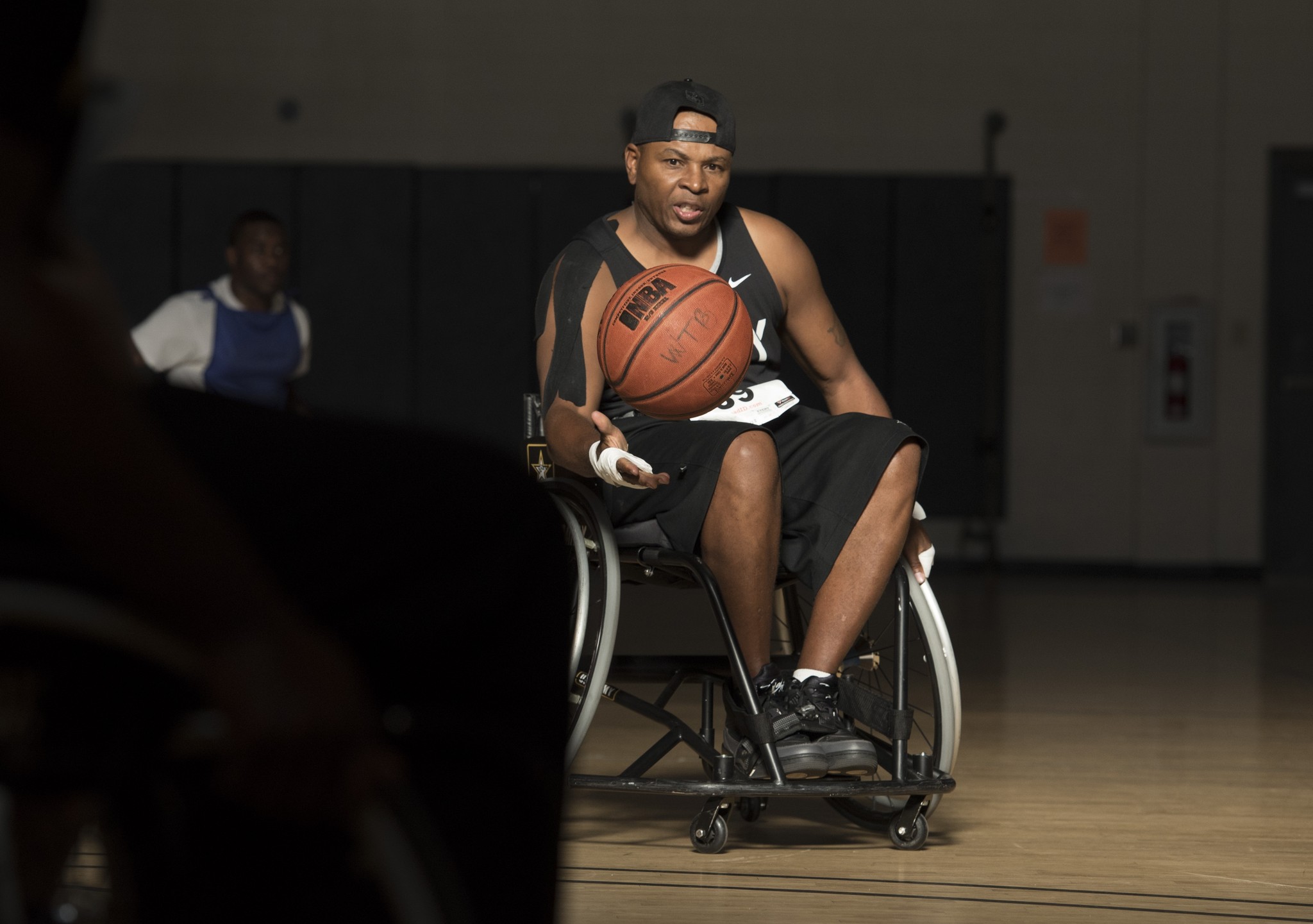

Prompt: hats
[{"left": 629, "top": 78, "right": 737, "bottom": 157}]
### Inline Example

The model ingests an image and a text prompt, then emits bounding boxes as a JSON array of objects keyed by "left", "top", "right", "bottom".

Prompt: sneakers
[
  {"left": 788, "top": 675, "right": 878, "bottom": 776},
  {"left": 721, "top": 659, "right": 830, "bottom": 781}
]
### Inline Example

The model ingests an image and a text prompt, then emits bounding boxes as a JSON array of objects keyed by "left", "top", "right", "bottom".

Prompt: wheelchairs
[{"left": 517, "top": 391, "right": 965, "bottom": 852}]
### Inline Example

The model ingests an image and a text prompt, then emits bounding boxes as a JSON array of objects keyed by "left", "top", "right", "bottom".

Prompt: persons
[
  {"left": 0, "top": 1, "right": 408, "bottom": 924},
  {"left": 527, "top": 75, "right": 937, "bottom": 784},
  {"left": 125, "top": 208, "right": 312, "bottom": 401}
]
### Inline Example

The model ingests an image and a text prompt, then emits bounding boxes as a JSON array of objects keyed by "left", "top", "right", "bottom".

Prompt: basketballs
[{"left": 596, "top": 264, "right": 752, "bottom": 422}]
